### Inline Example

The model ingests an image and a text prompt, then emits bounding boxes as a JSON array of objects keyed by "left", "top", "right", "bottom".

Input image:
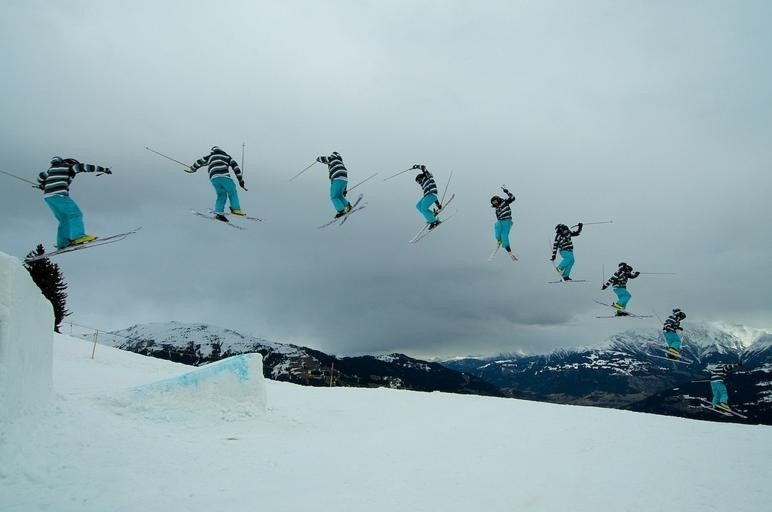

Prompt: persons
[
  {"left": 489, "top": 186, "right": 515, "bottom": 254},
  {"left": 662, "top": 306, "right": 686, "bottom": 359},
  {"left": 602, "top": 262, "right": 641, "bottom": 315},
  {"left": 37, "top": 157, "right": 112, "bottom": 251},
  {"left": 317, "top": 150, "right": 351, "bottom": 219},
  {"left": 548, "top": 223, "right": 584, "bottom": 280},
  {"left": 410, "top": 163, "right": 444, "bottom": 230},
  {"left": 190, "top": 144, "right": 246, "bottom": 222},
  {"left": 711, "top": 359, "right": 743, "bottom": 411}
]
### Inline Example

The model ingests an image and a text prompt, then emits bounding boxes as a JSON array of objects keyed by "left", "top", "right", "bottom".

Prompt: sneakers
[
  {"left": 497, "top": 240, "right": 573, "bottom": 283},
  {"left": 611, "top": 303, "right": 730, "bottom": 410},
  {"left": 72, "top": 234, "right": 98, "bottom": 246},
  {"left": 332, "top": 203, "right": 352, "bottom": 219},
  {"left": 427, "top": 219, "right": 441, "bottom": 232},
  {"left": 230, "top": 208, "right": 246, "bottom": 215}
]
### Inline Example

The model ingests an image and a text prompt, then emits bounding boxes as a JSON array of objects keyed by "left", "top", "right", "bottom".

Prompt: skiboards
[
  {"left": 701, "top": 400, "right": 747, "bottom": 420},
  {"left": 190, "top": 209, "right": 263, "bottom": 229},
  {"left": 25, "top": 227, "right": 141, "bottom": 262},
  {"left": 593, "top": 298, "right": 652, "bottom": 318},
  {"left": 316, "top": 194, "right": 368, "bottom": 227},
  {"left": 647, "top": 345, "right": 695, "bottom": 366},
  {"left": 487, "top": 240, "right": 503, "bottom": 261},
  {"left": 547, "top": 260, "right": 585, "bottom": 284},
  {"left": 409, "top": 195, "right": 455, "bottom": 242}
]
